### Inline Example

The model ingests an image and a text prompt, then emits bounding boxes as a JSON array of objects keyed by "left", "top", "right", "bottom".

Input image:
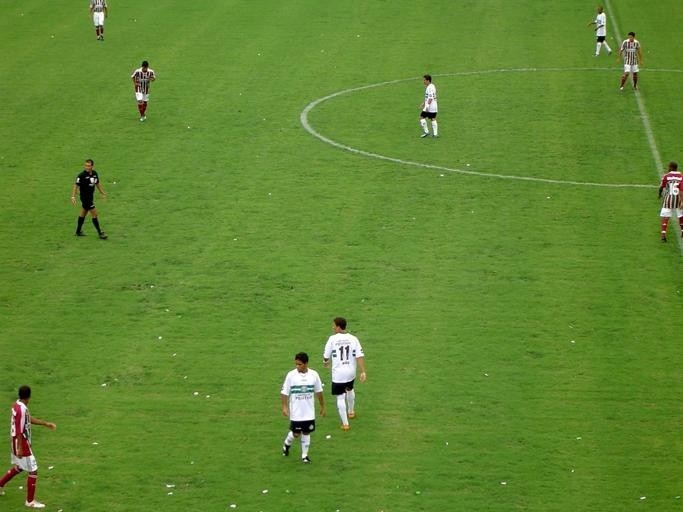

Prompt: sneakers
[
  {"left": 302, "top": 456, "right": 310, "bottom": 463},
  {"left": 281, "top": 445, "right": 290, "bottom": 456},
  {"left": 26, "top": 499, "right": 45, "bottom": 508}
]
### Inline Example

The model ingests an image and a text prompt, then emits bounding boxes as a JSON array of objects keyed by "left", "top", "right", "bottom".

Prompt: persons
[
  {"left": 1, "top": 386, "right": 57, "bottom": 509},
  {"left": 89, "top": 0, "right": 107, "bottom": 41},
  {"left": 658, "top": 162, "right": 683, "bottom": 242},
  {"left": 132, "top": 62, "right": 155, "bottom": 122},
  {"left": 281, "top": 353, "right": 326, "bottom": 464},
  {"left": 419, "top": 74, "right": 438, "bottom": 138},
  {"left": 323, "top": 318, "right": 366, "bottom": 430},
  {"left": 618, "top": 31, "right": 643, "bottom": 91},
  {"left": 71, "top": 159, "right": 109, "bottom": 240},
  {"left": 587, "top": 5, "right": 612, "bottom": 57}
]
